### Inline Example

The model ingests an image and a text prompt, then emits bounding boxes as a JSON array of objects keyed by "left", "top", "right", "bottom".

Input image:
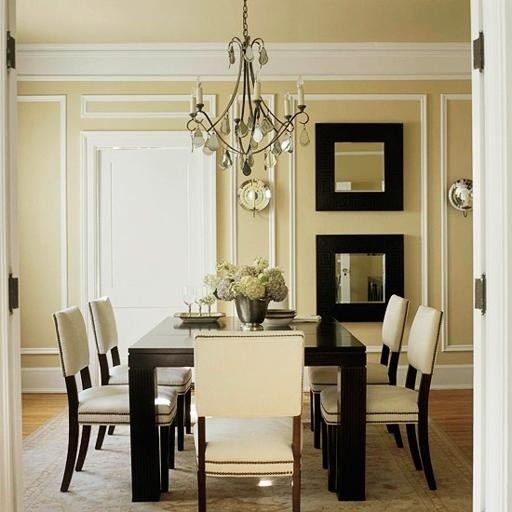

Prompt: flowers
[{"left": 200, "top": 256, "right": 289, "bottom": 303}]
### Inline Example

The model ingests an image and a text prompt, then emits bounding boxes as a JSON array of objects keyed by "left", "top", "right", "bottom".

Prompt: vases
[{"left": 235, "top": 297, "right": 272, "bottom": 331}]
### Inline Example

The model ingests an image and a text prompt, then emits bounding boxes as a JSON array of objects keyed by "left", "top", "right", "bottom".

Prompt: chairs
[
  {"left": 320, "top": 305, "right": 443, "bottom": 490},
  {"left": 306, "top": 295, "right": 410, "bottom": 449},
  {"left": 55, "top": 306, "right": 177, "bottom": 493},
  {"left": 89, "top": 296, "right": 192, "bottom": 452},
  {"left": 192, "top": 330, "right": 304, "bottom": 512}
]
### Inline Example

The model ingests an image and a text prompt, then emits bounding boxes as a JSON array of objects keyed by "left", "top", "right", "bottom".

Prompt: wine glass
[{"left": 183, "top": 285, "right": 216, "bottom": 317}]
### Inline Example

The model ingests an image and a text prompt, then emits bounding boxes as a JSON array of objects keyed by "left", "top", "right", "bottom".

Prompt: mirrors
[{"left": 315, "top": 123, "right": 404, "bottom": 324}]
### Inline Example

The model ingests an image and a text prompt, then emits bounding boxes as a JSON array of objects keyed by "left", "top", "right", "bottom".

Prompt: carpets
[{"left": 18, "top": 393, "right": 472, "bottom": 512}]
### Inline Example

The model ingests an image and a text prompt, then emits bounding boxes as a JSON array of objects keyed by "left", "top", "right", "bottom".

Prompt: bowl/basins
[{"left": 264, "top": 308, "right": 296, "bottom": 325}]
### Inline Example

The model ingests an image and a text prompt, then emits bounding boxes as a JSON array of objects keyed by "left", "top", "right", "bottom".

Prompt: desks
[{"left": 126, "top": 307, "right": 367, "bottom": 502}]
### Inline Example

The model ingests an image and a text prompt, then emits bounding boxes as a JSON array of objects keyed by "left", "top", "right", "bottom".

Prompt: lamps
[{"left": 185, "top": 0, "right": 311, "bottom": 218}]
